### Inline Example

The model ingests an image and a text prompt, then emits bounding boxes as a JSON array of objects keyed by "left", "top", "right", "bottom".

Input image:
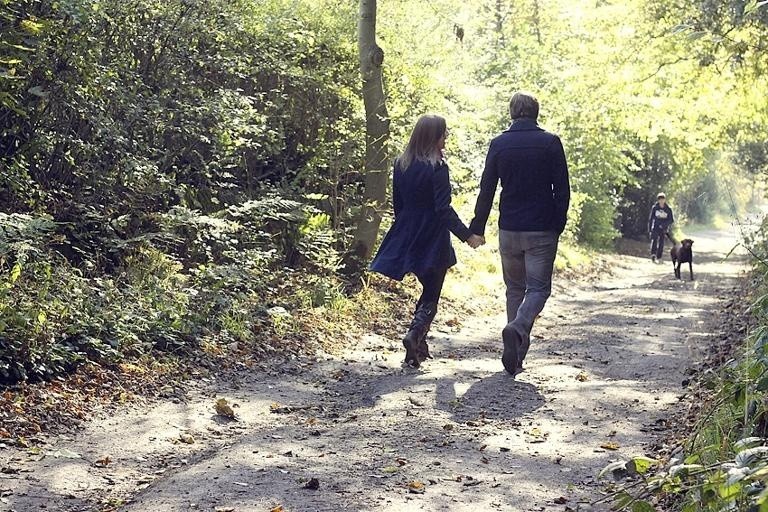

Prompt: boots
[{"left": 403, "top": 304, "right": 436, "bottom": 367}]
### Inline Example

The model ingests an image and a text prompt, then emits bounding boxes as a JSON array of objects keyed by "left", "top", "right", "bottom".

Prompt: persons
[
  {"left": 367, "top": 113, "right": 487, "bottom": 368},
  {"left": 467, "top": 90, "right": 570, "bottom": 376},
  {"left": 647, "top": 192, "right": 674, "bottom": 263}
]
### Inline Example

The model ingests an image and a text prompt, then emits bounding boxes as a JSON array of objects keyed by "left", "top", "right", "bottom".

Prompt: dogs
[{"left": 665, "top": 232, "right": 694, "bottom": 281}]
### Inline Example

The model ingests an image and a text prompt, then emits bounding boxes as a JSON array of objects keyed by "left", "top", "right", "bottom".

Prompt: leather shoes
[{"left": 502, "top": 326, "right": 522, "bottom": 374}]
